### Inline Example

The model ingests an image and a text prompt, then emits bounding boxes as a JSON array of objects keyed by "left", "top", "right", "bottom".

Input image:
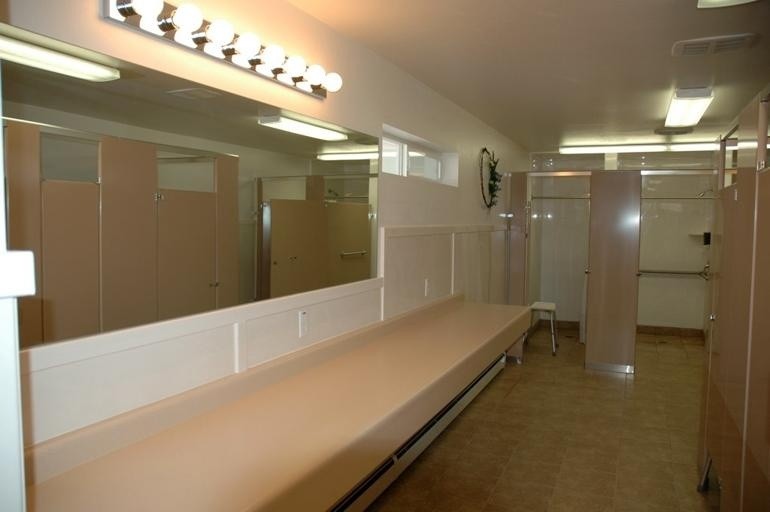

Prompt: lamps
[
  {"left": 0, "top": 34, "right": 120, "bottom": 82},
  {"left": 257, "top": 115, "right": 348, "bottom": 142},
  {"left": 664, "top": 87, "right": 714, "bottom": 127},
  {"left": 103, "top": 0, "right": 342, "bottom": 100},
  {"left": 559, "top": 142, "right": 721, "bottom": 154},
  {"left": 317, "top": 152, "right": 378, "bottom": 160}
]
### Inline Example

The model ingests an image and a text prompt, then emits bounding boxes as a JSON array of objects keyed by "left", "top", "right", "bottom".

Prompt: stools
[{"left": 523, "top": 302, "right": 559, "bottom": 356}]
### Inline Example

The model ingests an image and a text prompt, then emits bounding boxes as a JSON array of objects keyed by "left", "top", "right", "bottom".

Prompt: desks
[{"left": 23, "top": 292, "right": 531, "bottom": 512}]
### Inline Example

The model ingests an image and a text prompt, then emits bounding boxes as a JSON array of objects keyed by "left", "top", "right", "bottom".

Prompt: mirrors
[{"left": 0, "top": 34, "right": 379, "bottom": 350}]
